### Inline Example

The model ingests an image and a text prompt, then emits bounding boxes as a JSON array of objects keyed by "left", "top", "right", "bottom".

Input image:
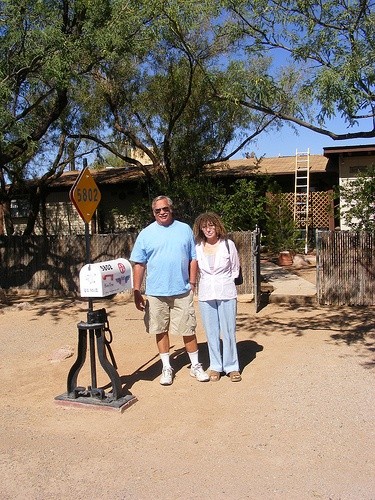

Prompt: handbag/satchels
[{"left": 224, "top": 238, "right": 243, "bottom": 285}]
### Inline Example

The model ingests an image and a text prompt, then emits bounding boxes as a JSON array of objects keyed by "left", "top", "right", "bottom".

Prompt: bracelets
[{"left": 189, "top": 282, "right": 197, "bottom": 288}]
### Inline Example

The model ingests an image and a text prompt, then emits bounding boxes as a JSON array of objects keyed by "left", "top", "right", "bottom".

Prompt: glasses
[{"left": 154, "top": 207, "right": 171, "bottom": 214}]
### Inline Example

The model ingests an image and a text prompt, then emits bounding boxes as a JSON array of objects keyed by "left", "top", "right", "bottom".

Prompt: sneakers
[
  {"left": 190, "top": 363, "right": 209, "bottom": 382},
  {"left": 159, "top": 366, "right": 174, "bottom": 385}
]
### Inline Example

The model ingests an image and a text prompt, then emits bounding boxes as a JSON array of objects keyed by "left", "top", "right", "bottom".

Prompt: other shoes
[
  {"left": 228, "top": 371, "right": 242, "bottom": 382},
  {"left": 209, "top": 370, "right": 220, "bottom": 382}
]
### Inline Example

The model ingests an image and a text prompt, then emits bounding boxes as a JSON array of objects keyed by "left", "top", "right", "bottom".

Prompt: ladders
[{"left": 292, "top": 147, "right": 310, "bottom": 255}]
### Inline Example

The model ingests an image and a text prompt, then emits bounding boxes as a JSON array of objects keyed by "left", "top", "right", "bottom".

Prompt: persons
[
  {"left": 129, "top": 195, "right": 210, "bottom": 385},
  {"left": 191, "top": 212, "right": 242, "bottom": 381}
]
